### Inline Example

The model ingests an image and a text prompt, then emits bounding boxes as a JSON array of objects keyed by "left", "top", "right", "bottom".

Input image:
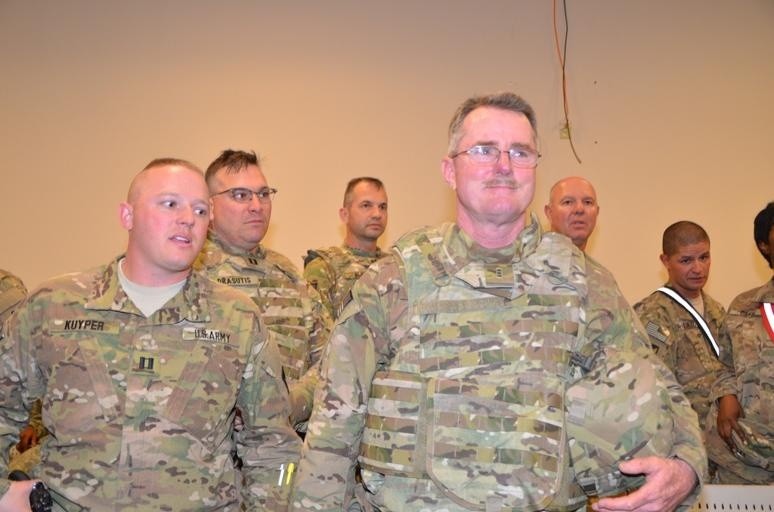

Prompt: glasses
[
  {"left": 449, "top": 141, "right": 542, "bottom": 167},
  {"left": 209, "top": 183, "right": 279, "bottom": 207}
]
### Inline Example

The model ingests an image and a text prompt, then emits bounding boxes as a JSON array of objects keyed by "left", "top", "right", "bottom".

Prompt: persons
[
  {"left": 1, "top": 269, "right": 51, "bottom": 481},
  {"left": 302, "top": 177, "right": 392, "bottom": 325},
  {"left": 287, "top": 92, "right": 710, "bottom": 512},
  {"left": 193, "top": 150, "right": 334, "bottom": 429},
  {"left": 0, "top": 157, "right": 303, "bottom": 512},
  {"left": 544, "top": 177, "right": 600, "bottom": 256},
  {"left": 702, "top": 201, "right": 774, "bottom": 485},
  {"left": 631, "top": 220, "right": 727, "bottom": 440}
]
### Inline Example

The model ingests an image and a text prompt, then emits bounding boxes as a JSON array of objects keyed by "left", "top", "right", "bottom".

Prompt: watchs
[{"left": 26, "top": 481, "right": 54, "bottom": 512}]
